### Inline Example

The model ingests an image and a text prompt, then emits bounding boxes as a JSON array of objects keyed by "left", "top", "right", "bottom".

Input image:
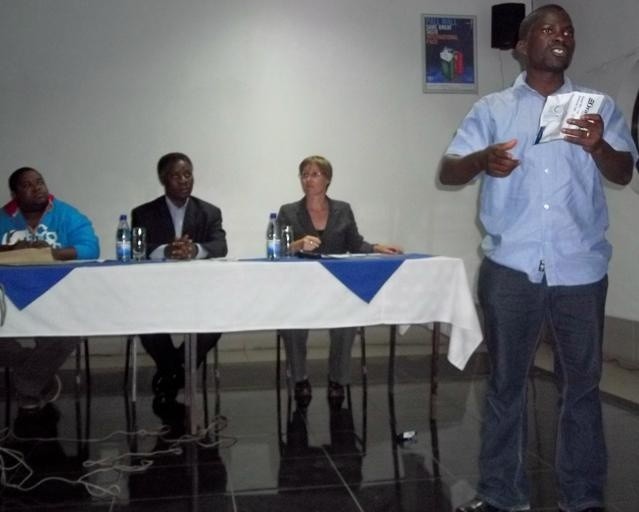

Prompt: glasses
[{"left": 300, "top": 171, "right": 324, "bottom": 178}]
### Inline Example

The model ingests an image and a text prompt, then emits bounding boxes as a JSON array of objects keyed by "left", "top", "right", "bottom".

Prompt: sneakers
[
  {"left": 42, "top": 373, "right": 62, "bottom": 403},
  {"left": 20, "top": 395, "right": 39, "bottom": 408}
]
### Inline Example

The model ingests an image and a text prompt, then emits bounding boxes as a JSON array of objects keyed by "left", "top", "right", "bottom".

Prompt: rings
[
  {"left": 586, "top": 131, "right": 591, "bottom": 138},
  {"left": 310, "top": 241, "right": 313, "bottom": 245}
]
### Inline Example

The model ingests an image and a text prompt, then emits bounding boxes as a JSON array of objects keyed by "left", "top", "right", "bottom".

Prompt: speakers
[{"left": 491, "top": 3, "right": 526, "bottom": 51}]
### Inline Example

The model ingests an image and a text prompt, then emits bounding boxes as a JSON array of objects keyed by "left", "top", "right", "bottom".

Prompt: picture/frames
[{"left": 422, "top": 15, "right": 480, "bottom": 95}]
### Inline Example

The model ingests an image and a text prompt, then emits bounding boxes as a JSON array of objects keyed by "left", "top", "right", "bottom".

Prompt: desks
[
  {"left": 107, "top": 420, "right": 457, "bottom": 511},
  {"left": 1, "top": 252, "right": 486, "bottom": 419}
]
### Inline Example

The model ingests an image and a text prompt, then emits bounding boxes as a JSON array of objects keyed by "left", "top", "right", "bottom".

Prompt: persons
[
  {"left": 270, "top": 394, "right": 376, "bottom": 507},
  {"left": 434, "top": 4, "right": 638, "bottom": 509},
  {"left": 276, "top": 155, "right": 405, "bottom": 412},
  {"left": 127, "top": 386, "right": 246, "bottom": 503},
  {"left": 130, "top": 152, "right": 228, "bottom": 411},
  {"left": 2, "top": 164, "right": 101, "bottom": 412}
]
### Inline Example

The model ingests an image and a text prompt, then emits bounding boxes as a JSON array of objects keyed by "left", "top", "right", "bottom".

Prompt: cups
[
  {"left": 279, "top": 223, "right": 292, "bottom": 260},
  {"left": 130, "top": 227, "right": 146, "bottom": 261}
]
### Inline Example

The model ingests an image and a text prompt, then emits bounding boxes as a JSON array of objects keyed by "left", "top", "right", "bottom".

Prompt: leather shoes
[
  {"left": 295, "top": 380, "right": 311, "bottom": 398},
  {"left": 457, "top": 497, "right": 500, "bottom": 512},
  {"left": 328, "top": 381, "right": 344, "bottom": 399},
  {"left": 153, "top": 366, "right": 185, "bottom": 407}
]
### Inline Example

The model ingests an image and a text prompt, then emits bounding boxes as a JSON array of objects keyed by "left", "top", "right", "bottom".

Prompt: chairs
[
  {"left": 121, "top": 331, "right": 222, "bottom": 404},
  {"left": 272, "top": 399, "right": 370, "bottom": 498},
  {"left": 123, "top": 404, "right": 231, "bottom": 500},
  {"left": 272, "top": 328, "right": 369, "bottom": 401},
  {"left": 2, "top": 339, "right": 93, "bottom": 400}
]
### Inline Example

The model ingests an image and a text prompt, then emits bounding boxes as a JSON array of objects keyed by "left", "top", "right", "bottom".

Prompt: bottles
[
  {"left": 116, "top": 215, "right": 132, "bottom": 263},
  {"left": 266, "top": 212, "right": 281, "bottom": 260}
]
